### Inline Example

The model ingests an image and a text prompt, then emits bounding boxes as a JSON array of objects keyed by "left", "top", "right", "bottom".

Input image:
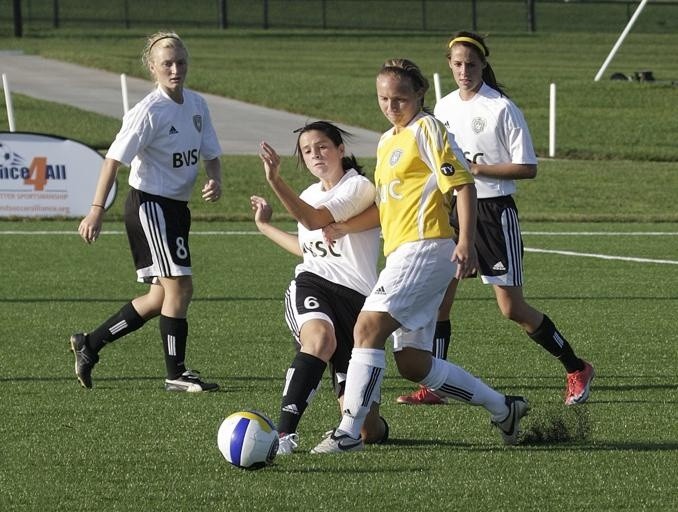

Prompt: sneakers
[
  {"left": 274, "top": 434, "right": 300, "bottom": 454},
  {"left": 163, "top": 370, "right": 219, "bottom": 393},
  {"left": 489, "top": 394, "right": 528, "bottom": 446},
  {"left": 396, "top": 386, "right": 447, "bottom": 406},
  {"left": 564, "top": 358, "right": 595, "bottom": 406},
  {"left": 69, "top": 333, "right": 99, "bottom": 390},
  {"left": 308, "top": 426, "right": 364, "bottom": 455}
]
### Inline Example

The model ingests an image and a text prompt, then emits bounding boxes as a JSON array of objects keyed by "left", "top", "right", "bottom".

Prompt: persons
[
  {"left": 249, "top": 118, "right": 389, "bottom": 455},
  {"left": 395, "top": 32, "right": 597, "bottom": 407},
  {"left": 69, "top": 31, "right": 224, "bottom": 393},
  {"left": 311, "top": 55, "right": 531, "bottom": 452}
]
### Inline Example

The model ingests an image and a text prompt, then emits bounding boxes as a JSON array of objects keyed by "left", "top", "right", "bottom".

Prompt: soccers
[{"left": 216, "top": 412, "right": 280, "bottom": 469}]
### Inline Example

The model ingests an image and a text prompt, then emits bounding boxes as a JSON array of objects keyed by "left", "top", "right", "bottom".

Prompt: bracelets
[{"left": 89, "top": 203, "right": 105, "bottom": 208}]
